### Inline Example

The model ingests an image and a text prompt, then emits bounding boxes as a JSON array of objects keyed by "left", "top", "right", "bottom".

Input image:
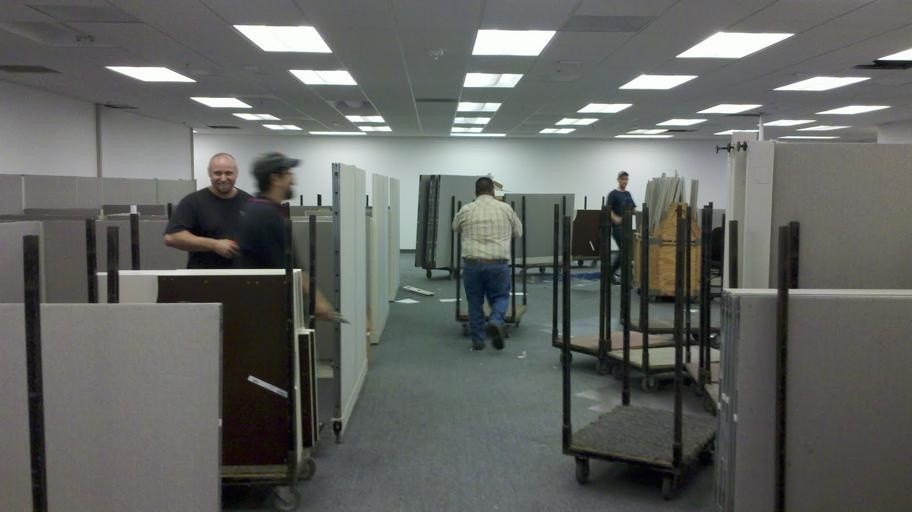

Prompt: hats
[{"left": 251, "top": 153, "right": 301, "bottom": 178}]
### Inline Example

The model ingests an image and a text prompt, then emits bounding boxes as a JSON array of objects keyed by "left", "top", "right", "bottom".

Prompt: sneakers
[
  {"left": 487, "top": 320, "right": 506, "bottom": 349},
  {"left": 611, "top": 270, "right": 617, "bottom": 284}
]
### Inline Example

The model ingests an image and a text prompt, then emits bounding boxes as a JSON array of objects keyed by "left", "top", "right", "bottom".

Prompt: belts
[{"left": 463, "top": 257, "right": 509, "bottom": 264}]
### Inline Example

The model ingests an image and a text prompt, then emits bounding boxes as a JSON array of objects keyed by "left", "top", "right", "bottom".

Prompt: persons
[
  {"left": 164, "top": 152, "right": 332, "bottom": 321},
  {"left": 607, "top": 172, "right": 637, "bottom": 287},
  {"left": 451, "top": 178, "right": 524, "bottom": 349}
]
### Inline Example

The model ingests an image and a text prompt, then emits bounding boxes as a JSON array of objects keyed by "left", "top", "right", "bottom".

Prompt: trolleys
[{"left": 85, "top": 212, "right": 325, "bottom": 511}]
